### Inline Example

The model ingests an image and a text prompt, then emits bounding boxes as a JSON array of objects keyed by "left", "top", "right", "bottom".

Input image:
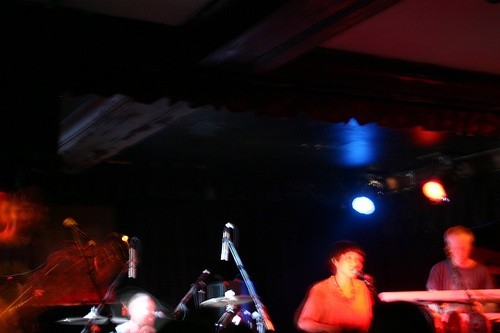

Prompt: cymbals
[
  {"left": 200, "top": 295, "right": 253, "bottom": 307},
  {"left": 58, "top": 316, "right": 127, "bottom": 326}
]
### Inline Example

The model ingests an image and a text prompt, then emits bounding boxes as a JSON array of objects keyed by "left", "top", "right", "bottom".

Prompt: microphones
[
  {"left": 221, "top": 222, "right": 237, "bottom": 262},
  {"left": 127, "top": 237, "right": 142, "bottom": 279},
  {"left": 172, "top": 269, "right": 211, "bottom": 318},
  {"left": 353, "top": 269, "right": 375, "bottom": 289}
]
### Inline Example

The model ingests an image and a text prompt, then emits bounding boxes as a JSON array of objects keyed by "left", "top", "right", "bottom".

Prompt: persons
[
  {"left": 426, "top": 226, "right": 500, "bottom": 333},
  {"left": 297, "top": 240, "right": 376, "bottom": 333},
  {"left": 110, "top": 292, "right": 157, "bottom": 333}
]
